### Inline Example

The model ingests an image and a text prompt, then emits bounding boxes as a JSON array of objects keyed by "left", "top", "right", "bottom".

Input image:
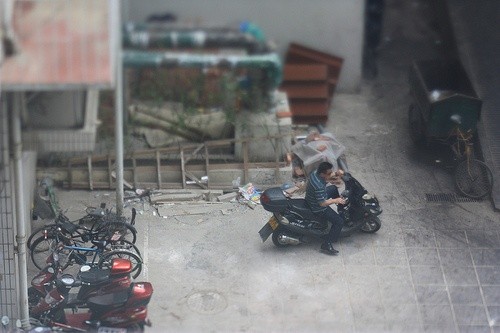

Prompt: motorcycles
[
  {"left": 28, "top": 238, "right": 155, "bottom": 332},
  {"left": 257, "top": 157, "right": 385, "bottom": 248}
]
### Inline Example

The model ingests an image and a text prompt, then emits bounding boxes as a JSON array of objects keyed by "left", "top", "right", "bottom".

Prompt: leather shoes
[{"left": 319, "top": 242, "right": 339, "bottom": 254}]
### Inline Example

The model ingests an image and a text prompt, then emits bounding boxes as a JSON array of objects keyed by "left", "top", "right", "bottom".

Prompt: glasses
[{"left": 327, "top": 170, "right": 333, "bottom": 176}]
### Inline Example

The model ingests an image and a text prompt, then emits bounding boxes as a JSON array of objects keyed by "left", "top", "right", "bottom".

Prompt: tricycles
[{"left": 405, "top": 59, "right": 495, "bottom": 199}]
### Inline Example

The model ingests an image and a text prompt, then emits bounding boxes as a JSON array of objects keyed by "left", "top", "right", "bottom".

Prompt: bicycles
[{"left": 27, "top": 203, "right": 144, "bottom": 280}]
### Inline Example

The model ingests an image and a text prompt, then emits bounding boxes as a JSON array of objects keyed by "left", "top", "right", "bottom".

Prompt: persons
[{"left": 304, "top": 162, "right": 347, "bottom": 255}]
